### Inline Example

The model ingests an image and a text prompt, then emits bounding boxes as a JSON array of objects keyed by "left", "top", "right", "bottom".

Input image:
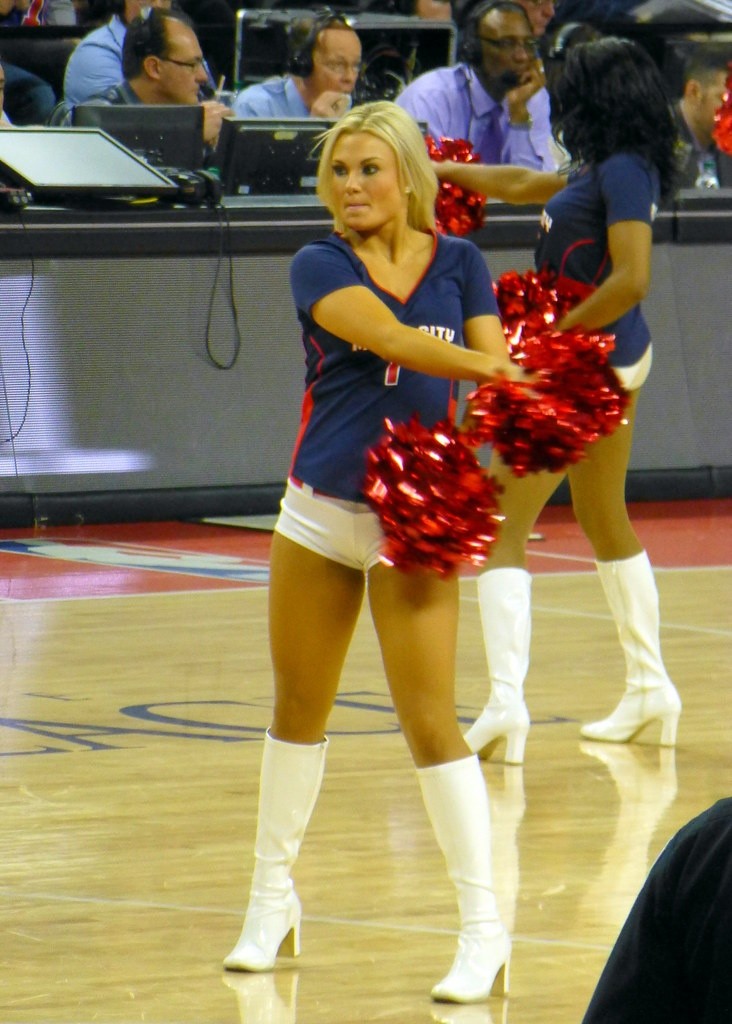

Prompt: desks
[{"left": 0, "top": 188, "right": 732, "bottom": 528}]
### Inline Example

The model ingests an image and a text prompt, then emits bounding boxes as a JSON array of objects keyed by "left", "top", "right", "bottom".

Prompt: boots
[
  {"left": 226, "top": 976, "right": 300, "bottom": 1024},
  {"left": 579, "top": 550, "right": 681, "bottom": 747},
  {"left": 581, "top": 744, "right": 677, "bottom": 910},
  {"left": 429, "top": 1006, "right": 505, "bottom": 1024},
  {"left": 461, "top": 567, "right": 533, "bottom": 765},
  {"left": 414, "top": 752, "right": 512, "bottom": 1002},
  {"left": 223, "top": 728, "right": 329, "bottom": 972}
]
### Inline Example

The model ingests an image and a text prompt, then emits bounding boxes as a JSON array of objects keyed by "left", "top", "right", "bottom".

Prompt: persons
[
  {"left": 231, "top": 11, "right": 361, "bottom": 117},
  {"left": 668, "top": 51, "right": 732, "bottom": 187},
  {"left": 60, "top": 6, "right": 234, "bottom": 149},
  {"left": 430, "top": 37, "right": 682, "bottom": 764},
  {"left": 65, "top": 0, "right": 215, "bottom": 105},
  {"left": 222, "top": 101, "right": 539, "bottom": 1004},
  {"left": 393, "top": 0, "right": 555, "bottom": 174}
]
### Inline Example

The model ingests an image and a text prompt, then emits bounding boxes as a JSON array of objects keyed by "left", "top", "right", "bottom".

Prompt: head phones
[
  {"left": 287, "top": 14, "right": 345, "bottom": 78},
  {"left": 460, "top": 0, "right": 527, "bottom": 65},
  {"left": 134, "top": 4, "right": 157, "bottom": 58},
  {"left": 162, "top": 170, "right": 223, "bottom": 207}
]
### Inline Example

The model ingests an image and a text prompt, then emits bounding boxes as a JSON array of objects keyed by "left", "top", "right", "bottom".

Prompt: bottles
[{"left": 695, "top": 161, "right": 720, "bottom": 188}]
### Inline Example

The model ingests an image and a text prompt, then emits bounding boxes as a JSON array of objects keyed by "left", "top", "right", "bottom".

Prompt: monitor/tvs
[
  {"left": 210, "top": 116, "right": 337, "bottom": 195},
  {"left": 71, "top": 104, "right": 206, "bottom": 170},
  {"left": 0, "top": 126, "right": 180, "bottom": 203}
]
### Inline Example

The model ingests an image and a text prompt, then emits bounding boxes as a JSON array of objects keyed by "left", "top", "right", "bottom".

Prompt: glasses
[
  {"left": 478, "top": 34, "right": 539, "bottom": 52},
  {"left": 160, "top": 54, "right": 206, "bottom": 75},
  {"left": 318, "top": 59, "right": 363, "bottom": 74}
]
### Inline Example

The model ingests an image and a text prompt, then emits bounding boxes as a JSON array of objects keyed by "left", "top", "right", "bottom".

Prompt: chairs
[{"left": 0, "top": 26, "right": 98, "bottom": 82}]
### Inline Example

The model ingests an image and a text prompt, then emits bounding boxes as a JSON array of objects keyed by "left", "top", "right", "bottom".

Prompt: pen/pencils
[{"left": 215, "top": 76, "right": 226, "bottom": 104}]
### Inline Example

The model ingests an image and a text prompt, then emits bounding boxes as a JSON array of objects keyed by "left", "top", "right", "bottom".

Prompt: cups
[{"left": 214, "top": 90, "right": 233, "bottom": 109}]
